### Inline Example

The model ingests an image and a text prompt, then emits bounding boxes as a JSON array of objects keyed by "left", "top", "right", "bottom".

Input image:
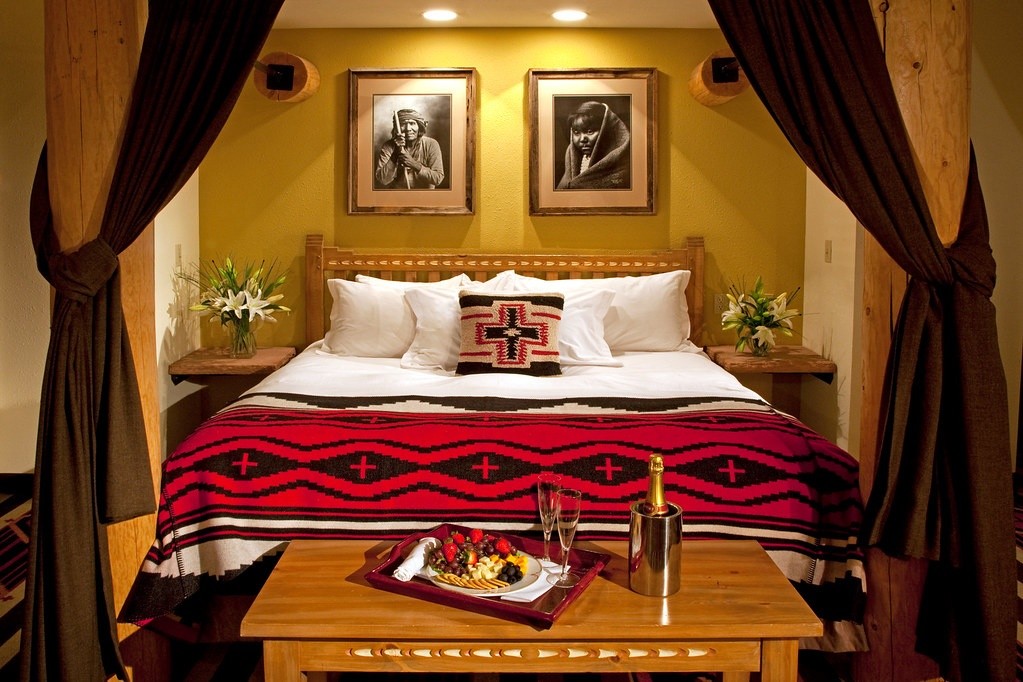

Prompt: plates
[
  {"left": 364, "top": 523, "right": 613, "bottom": 629},
  {"left": 427, "top": 550, "right": 542, "bottom": 596}
]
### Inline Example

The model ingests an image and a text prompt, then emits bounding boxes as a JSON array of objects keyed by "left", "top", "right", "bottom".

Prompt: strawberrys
[{"left": 441, "top": 528, "right": 510, "bottom": 565}]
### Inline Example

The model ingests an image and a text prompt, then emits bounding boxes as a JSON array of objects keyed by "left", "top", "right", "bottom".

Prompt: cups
[{"left": 628, "top": 500, "right": 682, "bottom": 598}]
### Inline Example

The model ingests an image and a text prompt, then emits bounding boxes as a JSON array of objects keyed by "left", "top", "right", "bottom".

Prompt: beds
[{"left": 115, "top": 234, "right": 873, "bottom": 655}]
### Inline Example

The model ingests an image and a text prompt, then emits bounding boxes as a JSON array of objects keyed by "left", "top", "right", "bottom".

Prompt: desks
[
  {"left": 168, "top": 346, "right": 295, "bottom": 411},
  {"left": 240, "top": 539, "right": 825, "bottom": 682},
  {"left": 705, "top": 344, "right": 838, "bottom": 420}
]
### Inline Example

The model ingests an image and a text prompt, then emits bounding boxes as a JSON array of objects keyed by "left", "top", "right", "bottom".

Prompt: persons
[
  {"left": 375, "top": 109, "right": 445, "bottom": 190},
  {"left": 556, "top": 101, "right": 631, "bottom": 189}
]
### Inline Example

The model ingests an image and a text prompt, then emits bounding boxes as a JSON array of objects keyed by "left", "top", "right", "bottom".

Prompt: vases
[
  {"left": 744, "top": 333, "right": 776, "bottom": 357},
  {"left": 227, "top": 323, "right": 257, "bottom": 359}
]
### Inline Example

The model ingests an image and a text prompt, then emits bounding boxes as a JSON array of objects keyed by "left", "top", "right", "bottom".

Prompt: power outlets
[
  {"left": 825, "top": 240, "right": 831, "bottom": 263},
  {"left": 714, "top": 293, "right": 728, "bottom": 314}
]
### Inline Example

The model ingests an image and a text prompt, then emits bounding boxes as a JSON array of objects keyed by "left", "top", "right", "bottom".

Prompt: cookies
[{"left": 436, "top": 573, "right": 510, "bottom": 590}]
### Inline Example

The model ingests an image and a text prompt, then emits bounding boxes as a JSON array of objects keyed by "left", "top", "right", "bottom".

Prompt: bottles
[{"left": 641, "top": 454, "right": 670, "bottom": 518}]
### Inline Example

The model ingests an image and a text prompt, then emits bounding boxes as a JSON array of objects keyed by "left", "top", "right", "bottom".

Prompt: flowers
[
  {"left": 720, "top": 271, "right": 820, "bottom": 355},
  {"left": 175, "top": 250, "right": 294, "bottom": 354}
]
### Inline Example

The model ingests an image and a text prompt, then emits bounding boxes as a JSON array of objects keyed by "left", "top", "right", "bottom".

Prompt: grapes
[{"left": 428, "top": 537, "right": 517, "bottom": 575}]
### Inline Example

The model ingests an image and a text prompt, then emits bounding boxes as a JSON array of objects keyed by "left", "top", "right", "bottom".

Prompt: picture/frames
[
  {"left": 348, "top": 67, "right": 475, "bottom": 216},
  {"left": 528, "top": 68, "right": 657, "bottom": 217}
]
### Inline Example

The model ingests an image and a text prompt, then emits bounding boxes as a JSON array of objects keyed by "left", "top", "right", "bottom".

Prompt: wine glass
[
  {"left": 546, "top": 488, "right": 582, "bottom": 588},
  {"left": 534, "top": 472, "right": 561, "bottom": 568}
]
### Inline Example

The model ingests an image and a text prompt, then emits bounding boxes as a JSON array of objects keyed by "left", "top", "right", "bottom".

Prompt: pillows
[
  {"left": 505, "top": 270, "right": 704, "bottom": 354},
  {"left": 357, "top": 272, "right": 464, "bottom": 287},
  {"left": 314, "top": 277, "right": 505, "bottom": 359},
  {"left": 455, "top": 290, "right": 565, "bottom": 377},
  {"left": 400, "top": 288, "right": 623, "bottom": 369}
]
